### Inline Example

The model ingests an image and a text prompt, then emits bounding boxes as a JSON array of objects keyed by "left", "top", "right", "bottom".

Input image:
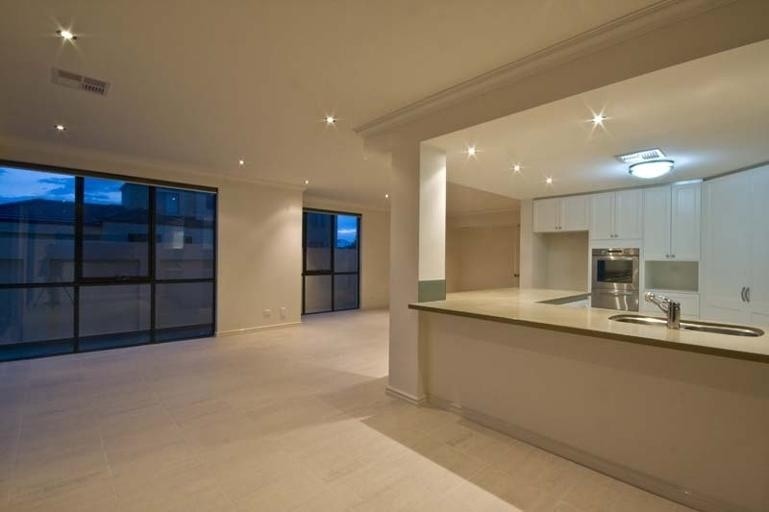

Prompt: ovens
[{"left": 591, "top": 248, "right": 640, "bottom": 312}]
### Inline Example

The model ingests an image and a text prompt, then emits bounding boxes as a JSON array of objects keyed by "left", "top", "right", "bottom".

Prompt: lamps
[{"left": 629, "top": 160, "right": 674, "bottom": 179}]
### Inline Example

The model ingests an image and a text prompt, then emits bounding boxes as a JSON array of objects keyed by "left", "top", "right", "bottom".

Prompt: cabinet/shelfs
[
  {"left": 589, "top": 189, "right": 642, "bottom": 236},
  {"left": 533, "top": 195, "right": 589, "bottom": 231},
  {"left": 700, "top": 165, "right": 768, "bottom": 326},
  {"left": 639, "top": 288, "right": 701, "bottom": 322},
  {"left": 642, "top": 180, "right": 701, "bottom": 261}
]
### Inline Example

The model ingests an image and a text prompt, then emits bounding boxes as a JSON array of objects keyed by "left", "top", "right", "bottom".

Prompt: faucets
[{"left": 644, "top": 291, "right": 681, "bottom": 329}]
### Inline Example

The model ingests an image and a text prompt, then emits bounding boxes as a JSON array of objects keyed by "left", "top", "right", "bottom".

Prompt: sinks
[
  {"left": 608, "top": 313, "right": 668, "bottom": 325},
  {"left": 685, "top": 321, "right": 765, "bottom": 337}
]
